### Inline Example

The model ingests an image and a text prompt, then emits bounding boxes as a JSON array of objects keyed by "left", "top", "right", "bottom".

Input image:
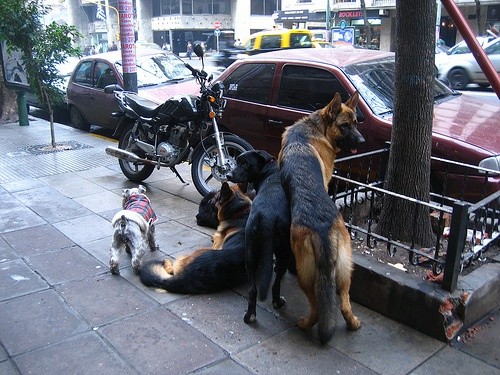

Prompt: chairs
[{"left": 97, "top": 68, "right": 118, "bottom": 88}]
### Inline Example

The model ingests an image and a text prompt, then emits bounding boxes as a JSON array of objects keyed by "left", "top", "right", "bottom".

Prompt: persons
[
  {"left": 233, "top": 38, "right": 241, "bottom": 47},
  {"left": 83, "top": 41, "right": 117, "bottom": 56},
  {"left": 187, "top": 41, "right": 192, "bottom": 59}
]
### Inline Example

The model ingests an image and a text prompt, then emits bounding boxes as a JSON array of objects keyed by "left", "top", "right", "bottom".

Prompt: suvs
[
  {"left": 239, "top": 30, "right": 314, "bottom": 48},
  {"left": 434, "top": 36, "right": 493, "bottom": 79}
]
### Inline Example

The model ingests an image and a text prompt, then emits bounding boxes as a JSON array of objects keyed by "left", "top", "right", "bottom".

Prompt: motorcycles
[{"left": 104, "top": 43, "right": 255, "bottom": 197}]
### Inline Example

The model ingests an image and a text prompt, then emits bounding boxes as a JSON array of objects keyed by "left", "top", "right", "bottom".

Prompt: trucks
[{"left": 1, "top": 34, "right": 97, "bottom": 118}]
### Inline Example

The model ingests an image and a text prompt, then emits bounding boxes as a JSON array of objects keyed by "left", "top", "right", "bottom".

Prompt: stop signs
[{"left": 214, "top": 21, "right": 220, "bottom": 28}]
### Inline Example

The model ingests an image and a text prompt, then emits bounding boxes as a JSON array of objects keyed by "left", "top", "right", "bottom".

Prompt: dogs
[
  {"left": 195, "top": 183, "right": 253, "bottom": 230},
  {"left": 276, "top": 88, "right": 362, "bottom": 350},
  {"left": 137, "top": 179, "right": 254, "bottom": 297},
  {"left": 107, "top": 183, "right": 161, "bottom": 277},
  {"left": 225, "top": 149, "right": 292, "bottom": 325}
]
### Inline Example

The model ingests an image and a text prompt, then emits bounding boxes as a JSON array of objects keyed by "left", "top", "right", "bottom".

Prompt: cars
[
  {"left": 440, "top": 37, "right": 500, "bottom": 89},
  {"left": 311, "top": 40, "right": 334, "bottom": 49},
  {"left": 206, "top": 46, "right": 499, "bottom": 208},
  {"left": 66, "top": 49, "right": 206, "bottom": 135}
]
